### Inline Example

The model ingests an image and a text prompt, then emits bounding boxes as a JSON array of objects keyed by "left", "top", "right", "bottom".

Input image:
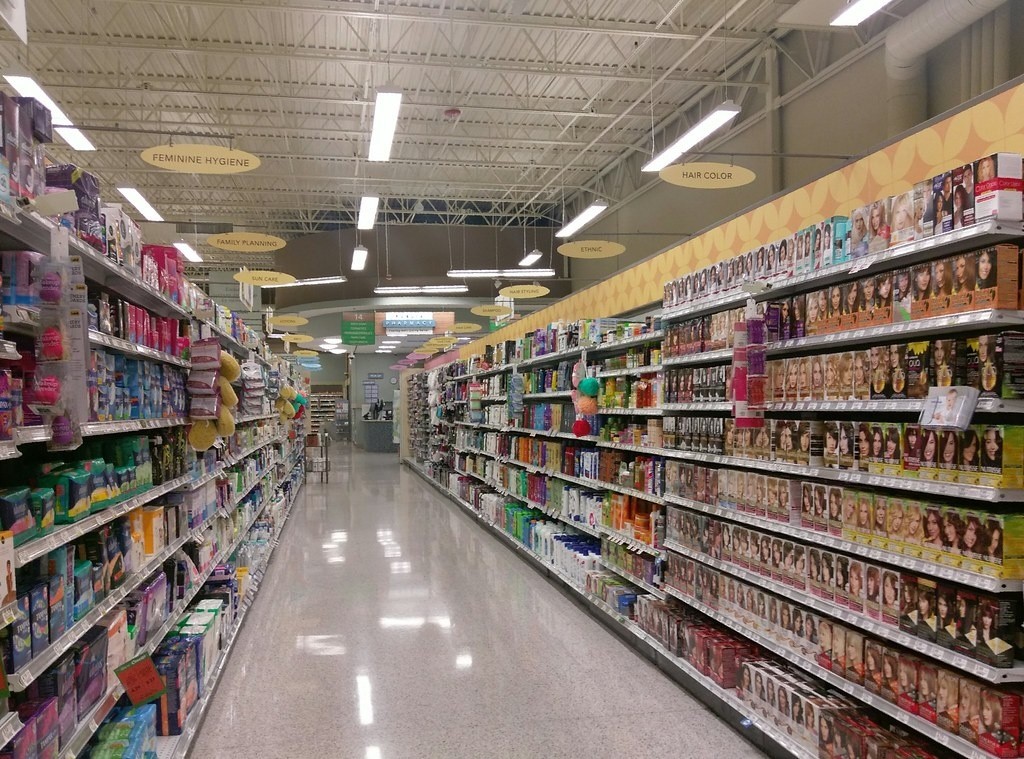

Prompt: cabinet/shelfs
[
  {"left": 401, "top": 78, "right": 1024, "bottom": 759},
  {"left": 0, "top": 191, "right": 311, "bottom": 759}
]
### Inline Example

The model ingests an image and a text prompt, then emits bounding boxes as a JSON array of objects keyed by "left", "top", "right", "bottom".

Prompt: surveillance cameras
[{"left": 348, "top": 352, "right": 355, "bottom": 359}]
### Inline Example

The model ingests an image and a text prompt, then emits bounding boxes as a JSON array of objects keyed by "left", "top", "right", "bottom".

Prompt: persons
[
  {"left": 636, "top": 245, "right": 1024, "bottom": 759},
  {"left": 845, "top": 153, "right": 999, "bottom": 258},
  {"left": 658, "top": 216, "right": 835, "bottom": 308},
  {"left": 583, "top": 574, "right": 608, "bottom": 601}
]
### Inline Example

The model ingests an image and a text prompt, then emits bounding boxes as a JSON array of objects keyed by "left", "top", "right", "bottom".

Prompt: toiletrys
[
  {"left": 426, "top": 316, "right": 662, "bottom": 591},
  {"left": 214, "top": 393, "right": 301, "bottom": 593}
]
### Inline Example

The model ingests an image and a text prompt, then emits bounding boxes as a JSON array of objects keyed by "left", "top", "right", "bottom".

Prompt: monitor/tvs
[{"left": 382, "top": 401, "right": 393, "bottom": 411}]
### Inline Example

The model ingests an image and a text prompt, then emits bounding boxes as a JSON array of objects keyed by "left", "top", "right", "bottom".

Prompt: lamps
[
  {"left": 357, "top": 156, "right": 379, "bottom": 230},
  {"left": 518, "top": 190, "right": 543, "bottom": 266},
  {"left": 350, "top": 230, "right": 369, "bottom": 271},
  {"left": 366, "top": 0, "right": 403, "bottom": 162},
  {"left": 374, "top": 215, "right": 469, "bottom": 293},
  {"left": 446, "top": 198, "right": 555, "bottom": 278},
  {"left": 641, "top": 0, "right": 742, "bottom": 173},
  {"left": 172, "top": 204, "right": 204, "bottom": 263},
  {"left": 4, "top": 40, "right": 98, "bottom": 151},
  {"left": 261, "top": 211, "right": 348, "bottom": 288},
  {"left": 554, "top": 152, "right": 609, "bottom": 238},
  {"left": 116, "top": 131, "right": 165, "bottom": 222},
  {"left": 828, "top": 0, "right": 892, "bottom": 28}
]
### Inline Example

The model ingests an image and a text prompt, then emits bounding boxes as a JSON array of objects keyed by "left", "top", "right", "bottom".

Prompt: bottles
[
  {"left": 235, "top": 514, "right": 276, "bottom": 570},
  {"left": 540, "top": 476, "right": 603, "bottom": 531},
  {"left": 458, "top": 476, "right": 511, "bottom": 527},
  {"left": 237, "top": 486, "right": 279, "bottom": 530},
  {"left": 564, "top": 447, "right": 600, "bottom": 480},
  {"left": 500, "top": 463, "right": 545, "bottom": 506},
  {"left": 603, "top": 492, "right": 666, "bottom": 550},
  {"left": 448, "top": 425, "right": 562, "bottom": 477},
  {"left": 426, "top": 461, "right": 462, "bottom": 494},
  {"left": 616, "top": 455, "right": 665, "bottom": 497},
  {"left": 598, "top": 416, "right": 654, "bottom": 448},
  {"left": 230, "top": 444, "right": 275, "bottom": 492},
  {"left": 515, "top": 328, "right": 548, "bottom": 362},
  {"left": 504, "top": 503, "right": 574, "bottom": 562},
  {"left": 589, "top": 312, "right": 661, "bottom": 409},
  {"left": 456, "top": 376, "right": 487, "bottom": 400},
  {"left": 553, "top": 533, "right": 601, "bottom": 585},
  {"left": 520, "top": 400, "right": 551, "bottom": 431}
]
